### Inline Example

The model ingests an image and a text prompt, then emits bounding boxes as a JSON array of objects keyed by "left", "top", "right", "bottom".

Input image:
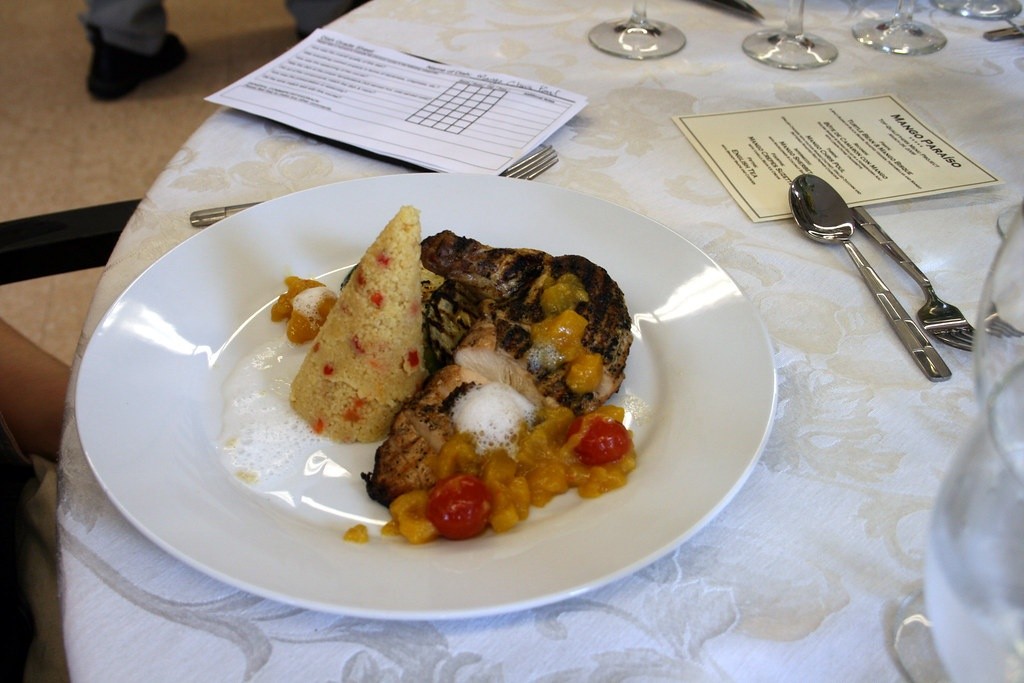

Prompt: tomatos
[
  {"left": 425, "top": 472, "right": 493, "bottom": 541},
  {"left": 563, "top": 413, "right": 629, "bottom": 467}
]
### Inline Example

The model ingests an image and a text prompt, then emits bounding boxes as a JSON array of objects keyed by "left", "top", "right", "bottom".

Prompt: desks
[{"left": 60, "top": 0, "right": 1024, "bottom": 683}]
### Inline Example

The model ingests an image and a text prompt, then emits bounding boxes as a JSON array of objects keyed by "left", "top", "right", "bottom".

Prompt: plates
[{"left": 73, "top": 169, "right": 777, "bottom": 617}]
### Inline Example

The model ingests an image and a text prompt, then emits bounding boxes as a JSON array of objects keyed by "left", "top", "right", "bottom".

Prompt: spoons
[{"left": 788, "top": 174, "right": 952, "bottom": 381}]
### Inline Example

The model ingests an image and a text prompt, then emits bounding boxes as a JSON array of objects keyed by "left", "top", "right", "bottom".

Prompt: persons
[
  {"left": 82, "top": 0, "right": 367, "bottom": 99},
  {"left": 0, "top": 317, "right": 71, "bottom": 683}
]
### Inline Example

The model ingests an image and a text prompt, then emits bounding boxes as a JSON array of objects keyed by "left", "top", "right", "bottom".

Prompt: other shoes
[
  {"left": 88, "top": 22, "right": 187, "bottom": 101},
  {"left": 296, "top": 0, "right": 368, "bottom": 41}
]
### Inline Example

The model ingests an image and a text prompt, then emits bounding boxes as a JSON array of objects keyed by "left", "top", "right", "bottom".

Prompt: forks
[
  {"left": 190, "top": 146, "right": 558, "bottom": 228},
  {"left": 849, "top": 206, "right": 986, "bottom": 352}
]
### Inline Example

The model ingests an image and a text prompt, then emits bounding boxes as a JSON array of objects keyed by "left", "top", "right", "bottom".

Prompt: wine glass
[
  {"left": 931, "top": 0, "right": 1022, "bottom": 20},
  {"left": 851, "top": 0, "right": 947, "bottom": 55},
  {"left": 892, "top": 183, "right": 1024, "bottom": 683},
  {"left": 741, "top": 0, "right": 838, "bottom": 71},
  {"left": 590, "top": 0, "right": 686, "bottom": 57}
]
